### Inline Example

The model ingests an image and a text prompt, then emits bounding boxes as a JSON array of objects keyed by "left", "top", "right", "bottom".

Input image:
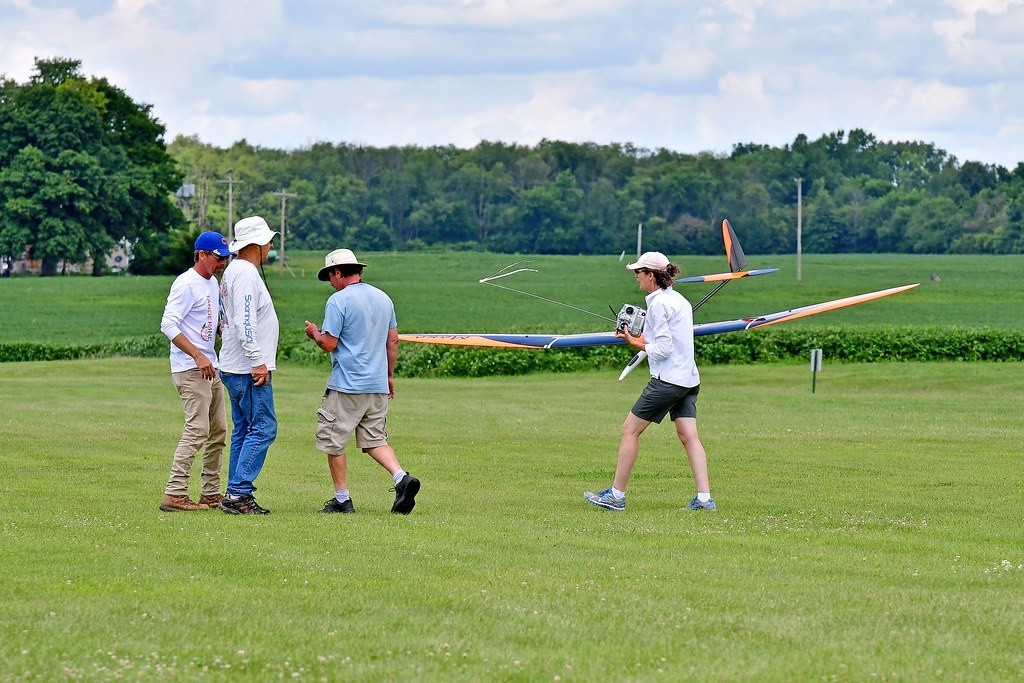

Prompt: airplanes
[{"left": 398, "top": 218, "right": 918, "bottom": 381}]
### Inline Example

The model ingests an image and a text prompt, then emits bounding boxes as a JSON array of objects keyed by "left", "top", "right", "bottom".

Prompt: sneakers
[
  {"left": 198, "top": 494, "right": 224, "bottom": 508},
  {"left": 318, "top": 497, "right": 354, "bottom": 514},
  {"left": 680, "top": 496, "right": 716, "bottom": 510},
  {"left": 584, "top": 487, "right": 626, "bottom": 511},
  {"left": 159, "top": 494, "right": 210, "bottom": 512},
  {"left": 389, "top": 472, "right": 421, "bottom": 515},
  {"left": 220, "top": 494, "right": 270, "bottom": 515}
]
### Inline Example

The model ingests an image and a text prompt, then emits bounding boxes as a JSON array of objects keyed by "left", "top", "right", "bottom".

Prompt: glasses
[
  {"left": 635, "top": 269, "right": 645, "bottom": 274},
  {"left": 270, "top": 240, "right": 273, "bottom": 245},
  {"left": 209, "top": 251, "right": 230, "bottom": 261}
]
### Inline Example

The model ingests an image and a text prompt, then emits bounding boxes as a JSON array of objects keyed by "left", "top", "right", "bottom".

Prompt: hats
[
  {"left": 229, "top": 216, "right": 282, "bottom": 252},
  {"left": 626, "top": 251, "right": 670, "bottom": 272},
  {"left": 317, "top": 248, "right": 368, "bottom": 281},
  {"left": 195, "top": 231, "right": 238, "bottom": 257}
]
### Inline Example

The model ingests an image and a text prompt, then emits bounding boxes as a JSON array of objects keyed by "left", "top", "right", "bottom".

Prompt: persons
[
  {"left": 305, "top": 249, "right": 421, "bottom": 516},
  {"left": 218, "top": 216, "right": 282, "bottom": 514},
  {"left": 160, "top": 232, "right": 228, "bottom": 513},
  {"left": 584, "top": 252, "right": 716, "bottom": 510}
]
